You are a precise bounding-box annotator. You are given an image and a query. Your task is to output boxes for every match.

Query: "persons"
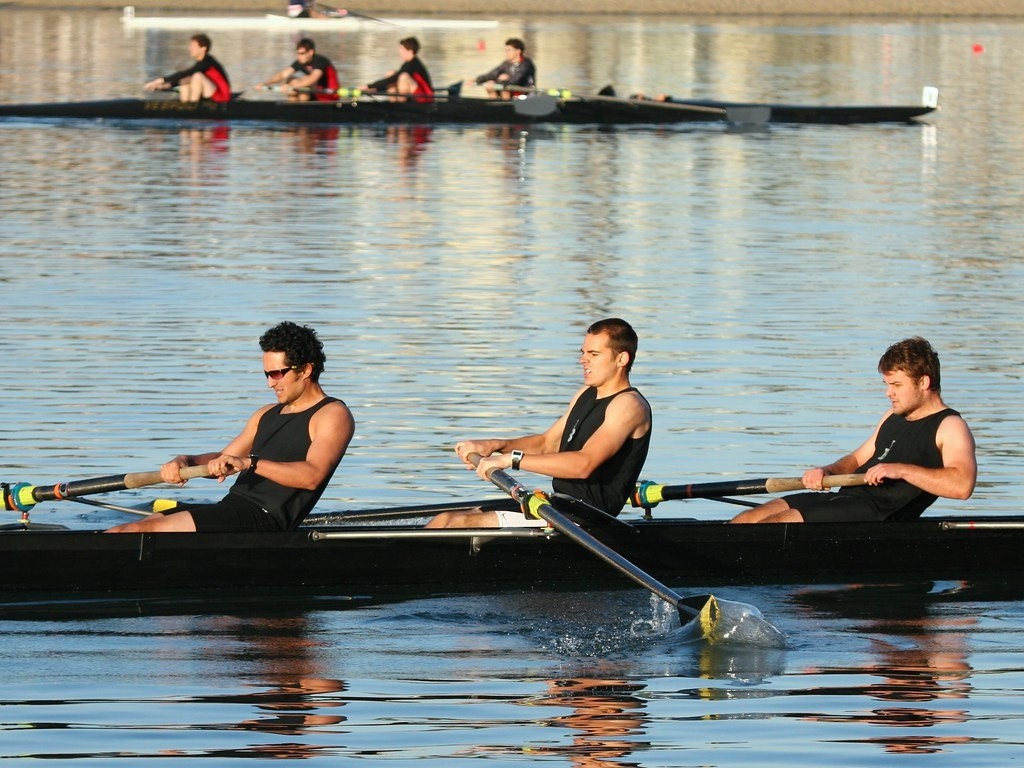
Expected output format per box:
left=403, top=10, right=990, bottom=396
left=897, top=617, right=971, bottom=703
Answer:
left=254, top=37, right=342, bottom=103
left=98, top=319, right=356, bottom=534
left=729, top=336, right=976, bottom=524
left=425, top=318, right=653, bottom=528
left=464, top=37, right=536, bottom=100
left=144, top=34, right=231, bottom=105
left=290, top=1, right=334, bottom=20
left=353, top=37, right=436, bottom=105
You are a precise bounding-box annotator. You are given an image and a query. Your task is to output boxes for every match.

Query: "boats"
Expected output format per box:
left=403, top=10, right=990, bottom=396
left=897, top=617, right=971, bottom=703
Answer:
left=0, top=516, right=1023, bottom=617
left=1, top=87, right=940, bottom=125
left=118, top=6, right=502, bottom=32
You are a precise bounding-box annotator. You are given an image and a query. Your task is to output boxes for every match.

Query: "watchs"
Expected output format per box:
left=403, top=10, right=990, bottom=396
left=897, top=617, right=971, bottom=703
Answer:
left=512, top=450, right=524, bottom=471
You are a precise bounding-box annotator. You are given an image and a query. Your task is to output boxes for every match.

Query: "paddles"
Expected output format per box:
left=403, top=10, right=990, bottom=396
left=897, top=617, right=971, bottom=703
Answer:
left=128, top=473, right=870, bottom=523
left=316, top=2, right=394, bottom=26
left=348, top=79, right=464, bottom=97
left=255, top=84, right=557, bottom=115
left=4, top=464, right=227, bottom=512
left=153, top=86, right=246, bottom=100
left=459, top=446, right=720, bottom=643
left=471, top=81, right=773, bottom=124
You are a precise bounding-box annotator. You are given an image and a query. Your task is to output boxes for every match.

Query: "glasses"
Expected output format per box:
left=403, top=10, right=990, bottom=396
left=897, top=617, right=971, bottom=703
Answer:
left=504, top=48, right=517, bottom=52
left=296, top=51, right=309, bottom=55
left=263, top=362, right=315, bottom=380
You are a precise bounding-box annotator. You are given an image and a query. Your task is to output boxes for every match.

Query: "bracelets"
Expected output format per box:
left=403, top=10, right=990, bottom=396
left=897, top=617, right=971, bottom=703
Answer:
left=247, top=454, right=261, bottom=474
